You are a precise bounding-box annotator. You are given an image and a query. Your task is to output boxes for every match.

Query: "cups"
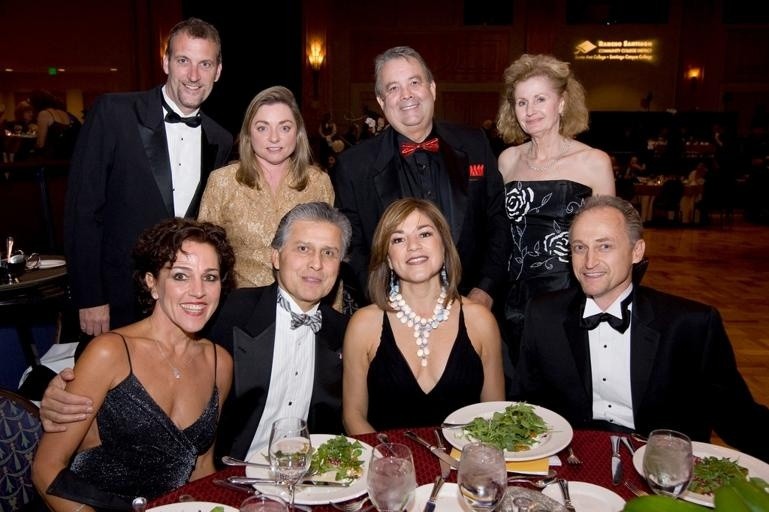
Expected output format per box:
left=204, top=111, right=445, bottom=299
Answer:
left=642, top=429, right=695, bottom=499
left=236, top=492, right=289, bottom=512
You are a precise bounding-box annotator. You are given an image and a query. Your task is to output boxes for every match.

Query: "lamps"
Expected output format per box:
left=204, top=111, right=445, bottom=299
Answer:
left=304, top=30, right=327, bottom=101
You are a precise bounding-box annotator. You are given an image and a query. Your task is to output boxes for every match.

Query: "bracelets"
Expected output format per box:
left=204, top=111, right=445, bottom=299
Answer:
left=75, top=504, right=86, bottom=512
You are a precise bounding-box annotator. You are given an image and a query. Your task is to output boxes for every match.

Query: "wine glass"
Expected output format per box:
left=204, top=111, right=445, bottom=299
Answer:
left=458, top=442, right=509, bottom=512
left=366, top=443, right=417, bottom=512
left=268, top=418, right=313, bottom=512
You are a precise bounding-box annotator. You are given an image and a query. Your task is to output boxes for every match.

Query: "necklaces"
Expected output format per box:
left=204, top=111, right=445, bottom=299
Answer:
left=148, top=316, right=197, bottom=379
left=388, top=284, right=453, bottom=367
left=525, top=135, right=573, bottom=172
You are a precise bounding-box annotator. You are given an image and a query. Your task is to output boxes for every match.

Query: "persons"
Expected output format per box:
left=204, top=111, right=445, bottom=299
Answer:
left=0, top=87, right=89, bottom=185
left=494, top=53, right=618, bottom=356
left=38, top=201, right=351, bottom=472
left=508, top=195, right=768, bottom=466
left=31, top=217, right=234, bottom=512
left=197, top=84, right=336, bottom=290
left=318, top=111, right=385, bottom=172
left=342, top=196, right=506, bottom=437
left=332, top=45, right=512, bottom=312
left=585, top=118, right=769, bottom=229
left=67, top=18, right=234, bottom=371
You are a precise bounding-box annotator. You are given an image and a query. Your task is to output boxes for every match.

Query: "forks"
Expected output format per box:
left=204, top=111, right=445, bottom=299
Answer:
left=566, top=443, right=585, bottom=465
left=329, top=490, right=373, bottom=511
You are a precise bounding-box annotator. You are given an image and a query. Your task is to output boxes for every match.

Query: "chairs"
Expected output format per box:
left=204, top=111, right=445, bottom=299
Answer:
left=1, top=387, right=49, bottom=512
left=614, top=122, right=769, bottom=229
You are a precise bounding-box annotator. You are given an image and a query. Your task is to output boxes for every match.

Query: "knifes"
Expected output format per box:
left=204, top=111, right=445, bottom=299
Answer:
left=623, top=479, right=649, bottom=498
left=433, top=428, right=451, bottom=479
left=558, top=478, right=576, bottom=512
left=608, top=434, right=625, bottom=487
left=423, top=476, right=446, bottom=512
left=377, top=431, right=399, bottom=458
left=404, top=430, right=459, bottom=469
left=227, top=474, right=350, bottom=488
left=212, top=476, right=313, bottom=512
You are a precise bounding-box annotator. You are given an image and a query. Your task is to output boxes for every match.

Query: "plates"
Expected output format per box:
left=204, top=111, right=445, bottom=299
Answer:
left=405, top=483, right=465, bottom=512
left=137, top=501, right=238, bottom=511
left=440, top=401, right=574, bottom=461
left=245, top=433, right=382, bottom=504
left=27, top=258, right=66, bottom=269
left=541, top=480, right=627, bottom=512
left=632, top=438, right=769, bottom=510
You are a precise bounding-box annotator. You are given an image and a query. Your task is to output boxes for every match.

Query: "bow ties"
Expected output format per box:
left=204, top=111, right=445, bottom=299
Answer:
left=291, top=310, right=322, bottom=333
left=165, top=111, right=202, bottom=127
left=400, top=138, right=439, bottom=156
left=580, top=298, right=630, bottom=333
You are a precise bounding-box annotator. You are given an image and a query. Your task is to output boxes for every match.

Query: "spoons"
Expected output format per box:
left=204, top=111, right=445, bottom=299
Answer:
left=509, top=476, right=559, bottom=487
